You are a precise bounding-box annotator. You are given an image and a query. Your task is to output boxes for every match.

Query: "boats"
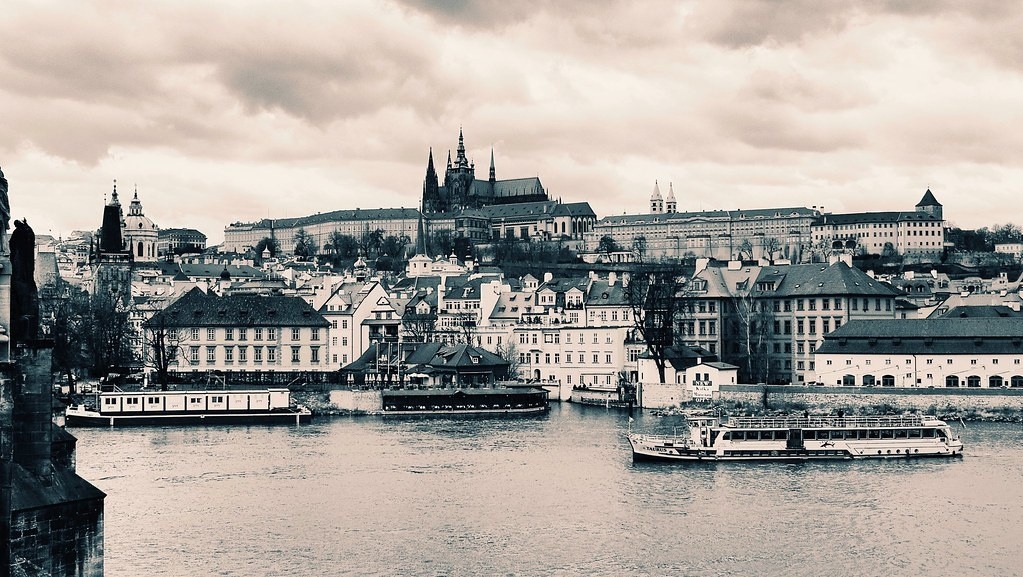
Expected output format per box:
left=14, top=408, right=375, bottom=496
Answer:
left=628, top=407, right=969, bottom=468
left=379, top=385, right=554, bottom=422
